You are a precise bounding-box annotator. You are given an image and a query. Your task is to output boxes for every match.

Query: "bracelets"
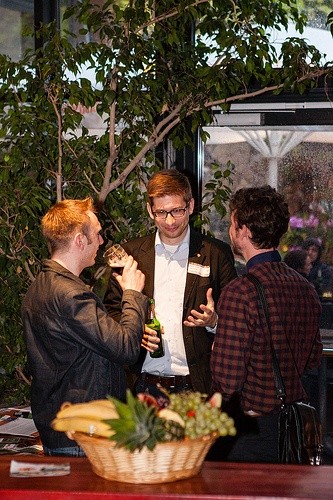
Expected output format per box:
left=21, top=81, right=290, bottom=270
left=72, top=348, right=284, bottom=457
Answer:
left=210, top=322, right=217, bottom=329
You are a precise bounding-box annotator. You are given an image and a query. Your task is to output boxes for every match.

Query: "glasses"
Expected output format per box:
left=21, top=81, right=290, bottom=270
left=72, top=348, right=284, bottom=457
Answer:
left=153, top=204, right=188, bottom=219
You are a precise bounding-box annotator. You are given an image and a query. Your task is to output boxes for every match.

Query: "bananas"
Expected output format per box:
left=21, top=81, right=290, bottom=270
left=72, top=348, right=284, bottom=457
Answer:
left=51, top=399, right=121, bottom=436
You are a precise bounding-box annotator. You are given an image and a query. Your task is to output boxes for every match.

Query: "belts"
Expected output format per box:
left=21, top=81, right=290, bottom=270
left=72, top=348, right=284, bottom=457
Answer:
left=143, top=374, right=191, bottom=388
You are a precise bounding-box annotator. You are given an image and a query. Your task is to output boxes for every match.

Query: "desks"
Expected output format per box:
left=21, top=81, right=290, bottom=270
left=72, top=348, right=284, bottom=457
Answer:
left=0, top=454, right=333, bottom=500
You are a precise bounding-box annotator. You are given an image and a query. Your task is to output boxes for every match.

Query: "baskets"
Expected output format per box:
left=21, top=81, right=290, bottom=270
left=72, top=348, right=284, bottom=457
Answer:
left=61, top=391, right=221, bottom=484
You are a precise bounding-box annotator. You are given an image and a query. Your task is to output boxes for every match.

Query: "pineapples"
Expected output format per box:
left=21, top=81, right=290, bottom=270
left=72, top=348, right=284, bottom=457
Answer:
left=103, top=387, right=186, bottom=452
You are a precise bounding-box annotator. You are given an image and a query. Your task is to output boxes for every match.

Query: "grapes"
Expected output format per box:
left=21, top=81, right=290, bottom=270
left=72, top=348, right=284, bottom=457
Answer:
left=167, top=391, right=237, bottom=440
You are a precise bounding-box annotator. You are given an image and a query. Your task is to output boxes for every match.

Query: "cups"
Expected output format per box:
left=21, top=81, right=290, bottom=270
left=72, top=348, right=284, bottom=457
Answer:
left=102, top=244, right=129, bottom=276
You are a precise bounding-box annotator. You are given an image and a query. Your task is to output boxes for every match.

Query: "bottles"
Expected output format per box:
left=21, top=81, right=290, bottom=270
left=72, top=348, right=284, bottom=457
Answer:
left=144, top=298, right=165, bottom=358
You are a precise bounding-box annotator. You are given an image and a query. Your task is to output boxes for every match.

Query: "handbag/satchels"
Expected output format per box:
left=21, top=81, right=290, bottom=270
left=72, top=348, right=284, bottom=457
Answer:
left=278, top=403, right=324, bottom=466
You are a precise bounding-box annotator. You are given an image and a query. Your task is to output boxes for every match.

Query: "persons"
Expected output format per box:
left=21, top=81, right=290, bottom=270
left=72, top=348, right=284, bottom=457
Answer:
left=103, top=169, right=240, bottom=462
left=209, top=185, right=323, bottom=466
left=21, top=196, right=149, bottom=457
left=285, top=239, right=329, bottom=296
